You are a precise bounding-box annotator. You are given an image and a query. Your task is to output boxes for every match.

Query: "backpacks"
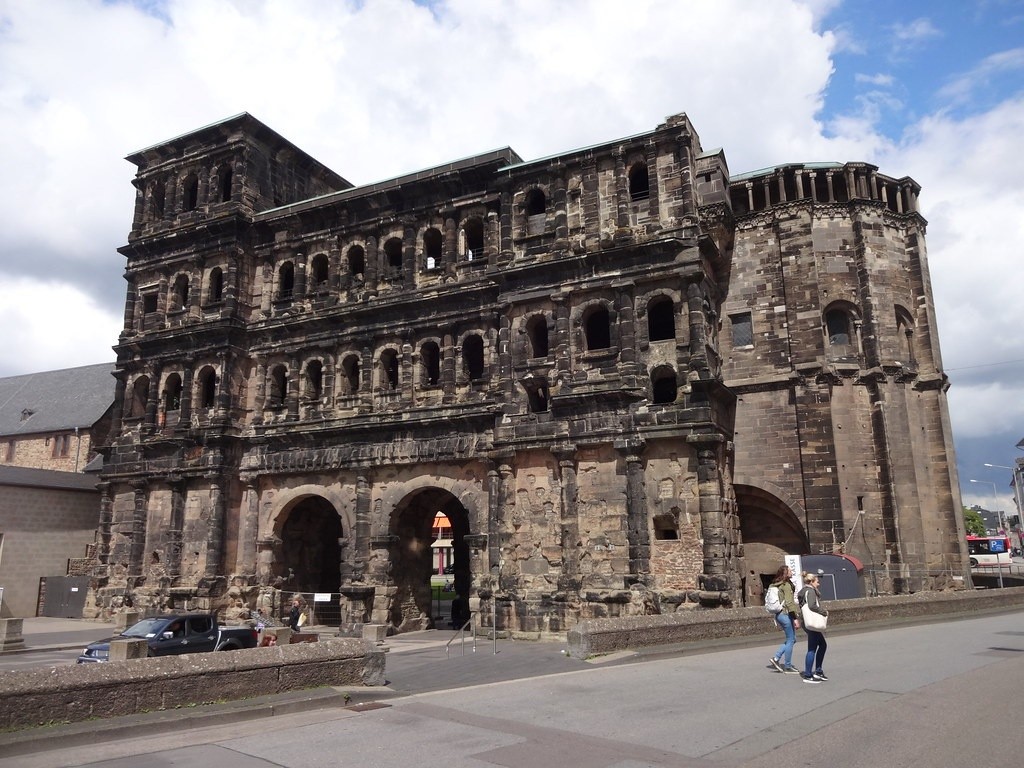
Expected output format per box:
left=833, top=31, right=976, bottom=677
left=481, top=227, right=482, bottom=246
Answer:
left=765, top=587, right=793, bottom=613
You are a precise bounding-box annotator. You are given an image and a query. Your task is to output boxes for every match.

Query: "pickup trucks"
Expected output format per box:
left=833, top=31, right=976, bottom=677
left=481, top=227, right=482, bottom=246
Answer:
left=77, top=610, right=257, bottom=665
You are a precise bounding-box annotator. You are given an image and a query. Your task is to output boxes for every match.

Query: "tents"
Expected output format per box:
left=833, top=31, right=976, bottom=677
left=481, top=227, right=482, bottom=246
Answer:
left=801, top=553, right=866, bottom=600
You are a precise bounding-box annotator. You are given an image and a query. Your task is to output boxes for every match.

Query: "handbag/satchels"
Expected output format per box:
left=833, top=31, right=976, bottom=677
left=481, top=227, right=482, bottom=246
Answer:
left=801, top=590, right=829, bottom=632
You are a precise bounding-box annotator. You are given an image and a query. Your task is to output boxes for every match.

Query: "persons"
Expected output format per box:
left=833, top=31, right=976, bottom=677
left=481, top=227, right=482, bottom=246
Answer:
left=173, top=619, right=184, bottom=639
left=258, top=634, right=277, bottom=647
left=171, top=397, right=179, bottom=411
left=797, top=570, right=829, bottom=683
left=769, top=564, right=800, bottom=675
left=288, top=595, right=303, bottom=634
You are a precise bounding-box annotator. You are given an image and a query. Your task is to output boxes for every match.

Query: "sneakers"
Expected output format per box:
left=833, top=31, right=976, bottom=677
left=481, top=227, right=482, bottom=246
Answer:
left=783, top=666, right=800, bottom=675
left=770, top=657, right=783, bottom=673
left=802, top=675, right=821, bottom=684
left=813, top=671, right=828, bottom=681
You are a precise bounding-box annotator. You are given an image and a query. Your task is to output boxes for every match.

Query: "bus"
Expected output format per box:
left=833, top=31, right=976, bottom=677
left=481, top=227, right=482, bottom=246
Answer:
left=966, top=534, right=1013, bottom=569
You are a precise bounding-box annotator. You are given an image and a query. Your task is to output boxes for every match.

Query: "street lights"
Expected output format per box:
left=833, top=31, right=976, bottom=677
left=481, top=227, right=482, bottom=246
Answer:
left=969, top=479, right=1005, bottom=535
left=983, top=462, right=1024, bottom=529
